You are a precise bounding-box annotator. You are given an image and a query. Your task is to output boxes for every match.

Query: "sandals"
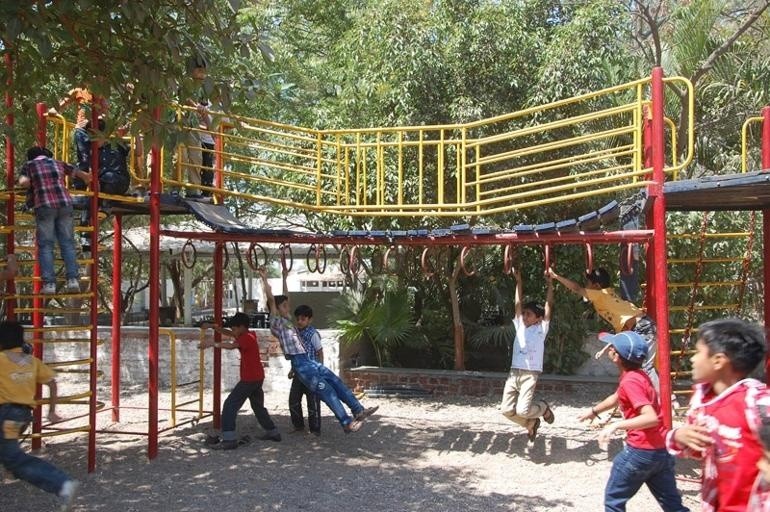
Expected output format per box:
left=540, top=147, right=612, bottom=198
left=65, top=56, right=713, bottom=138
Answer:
left=527, top=418, right=540, bottom=442
left=540, top=400, right=554, bottom=424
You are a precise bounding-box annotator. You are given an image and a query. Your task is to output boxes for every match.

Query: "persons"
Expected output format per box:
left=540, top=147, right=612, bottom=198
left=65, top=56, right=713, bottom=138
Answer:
left=574, top=330, right=691, bottom=512
left=196, top=311, right=282, bottom=451
left=614, top=192, right=646, bottom=305
left=18, top=145, right=102, bottom=296
left=543, top=265, right=681, bottom=419
left=500, top=262, right=555, bottom=442
left=49, top=82, right=135, bottom=190
left=194, top=108, right=217, bottom=198
left=0, top=319, right=82, bottom=512
left=664, top=317, right=769, bottom=512
left=285, top=304, right=324, bottom=439
left=72, top=119, right=132, bottom=253
left=170, top=57, right=216, bottom=197
left=258, top=263, right=380, bottom=435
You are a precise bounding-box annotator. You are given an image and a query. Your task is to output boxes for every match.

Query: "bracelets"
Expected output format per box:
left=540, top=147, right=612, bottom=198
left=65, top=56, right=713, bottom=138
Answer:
left=592, top=407, right=602, bottom=420
left=214, top=341, right=219, bottom=348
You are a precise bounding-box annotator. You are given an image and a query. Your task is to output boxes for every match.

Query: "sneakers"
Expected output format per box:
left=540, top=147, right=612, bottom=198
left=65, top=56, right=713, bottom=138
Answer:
left=65, top=278, right=79, bottom=288
left=305, top=432, right=320, bottom=440
left=343, top=421, right=363, bottom=432
left=209, top=440, right=238, bottom=450
left=258, top=433, right=281, bottom=442
left=60, top=480, right=81, bottom=511
left=289, top=429, right=304, bottom=435
left=40, top=283, right=55, bottom=294
left=356, top=405, right=379, bottom=420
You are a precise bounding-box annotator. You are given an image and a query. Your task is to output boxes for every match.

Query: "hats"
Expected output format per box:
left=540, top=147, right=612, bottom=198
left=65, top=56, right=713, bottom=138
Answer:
left=598, top=331, right=648, bottom=364
